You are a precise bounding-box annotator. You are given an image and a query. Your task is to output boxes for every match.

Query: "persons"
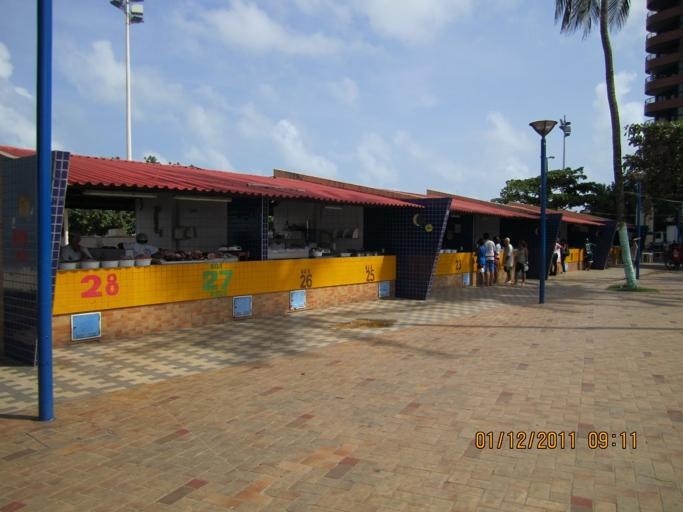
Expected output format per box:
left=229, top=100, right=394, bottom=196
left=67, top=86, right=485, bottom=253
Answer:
left=511, top=239, right=528, bottom=287
left=503, top=237, right=513, bottom=283
left=482, top=231, right=495, bottom=287
left=646, top=240, right=682, bottom=270
left=117, top=232, right=158, bottom=258
left=473, top=238, right=485, bottom=286
left=59, top=231, right=93, bottom=262
left=548, top=236, right=569, bottom=275
left=493, top=236, right=500, bottom=284
left=579, top=237, right=595, bottom=271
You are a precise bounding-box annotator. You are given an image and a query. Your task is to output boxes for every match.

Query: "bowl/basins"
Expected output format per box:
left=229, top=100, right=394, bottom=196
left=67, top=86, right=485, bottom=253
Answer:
left=79, top=261, right=99, bottom=269
left=118, top=259, right=135, bottom=267
left=439, top=249, right=457, bottom=254
left=339, top=252, right=352, bottom=257
left=135, top=259, right=152, bottom=266
left=100, top=261, right=119, bottom=267
left=312, top=252, right=322, bottom=257
left=58, top=262, right=76, bottom=270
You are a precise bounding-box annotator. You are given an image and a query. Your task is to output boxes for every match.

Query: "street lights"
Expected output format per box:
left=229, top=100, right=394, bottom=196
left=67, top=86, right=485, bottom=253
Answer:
left=559, top=116, right=571, bottom=169
left=528, top=120, right=558, bottom=304
left=631, top=172, right=648, bottom=280
left=111, top=0, right=144, bottom=161
